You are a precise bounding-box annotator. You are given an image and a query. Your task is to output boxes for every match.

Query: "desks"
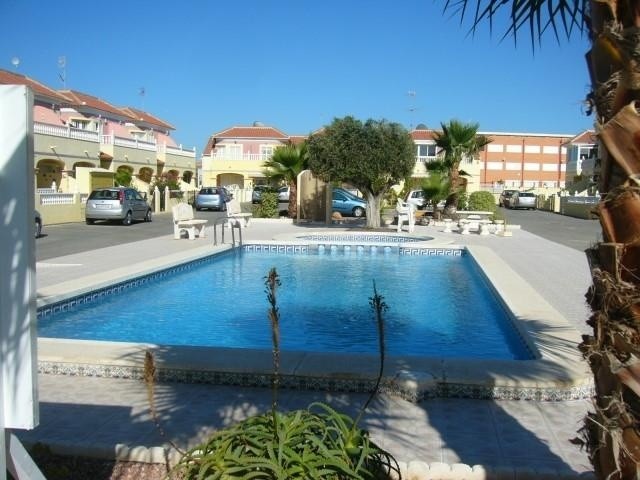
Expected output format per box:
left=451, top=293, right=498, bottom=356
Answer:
left=455, top=210, right=494, bottom=231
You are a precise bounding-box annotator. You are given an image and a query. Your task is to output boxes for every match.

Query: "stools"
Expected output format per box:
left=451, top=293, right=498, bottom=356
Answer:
left=443, top=219, right=504, bottom=235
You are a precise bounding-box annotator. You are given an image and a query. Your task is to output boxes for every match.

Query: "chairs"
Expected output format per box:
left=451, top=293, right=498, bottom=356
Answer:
left=171, top=203, right=208, bottom=240
left=226, top=198, right=253, bottom=228
left=396, top=198, right=415, bottom=234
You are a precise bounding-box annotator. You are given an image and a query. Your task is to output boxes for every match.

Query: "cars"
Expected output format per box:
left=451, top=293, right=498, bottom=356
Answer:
left=406, top=190, right=469, bottom=211
left=85, top=187, right=151, bottom=225
left=196, top=187, right=233, bottom=212
left=35, top=211, right=42, bottom=239
left=331, top=188, right=367, bottom=217
left=276, top=185, right=290, bottom=201
left=252, top=185, right=273, bottom=204
left=508, top=191, right=537, bottom=210
left=499, top=190, right=519, bottom=208
